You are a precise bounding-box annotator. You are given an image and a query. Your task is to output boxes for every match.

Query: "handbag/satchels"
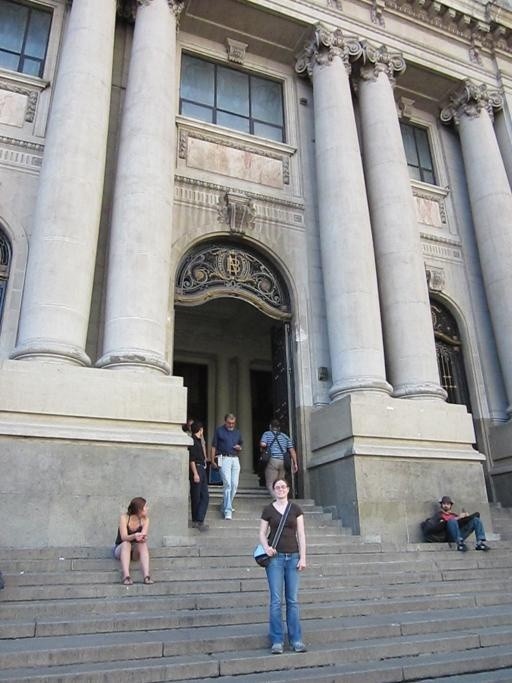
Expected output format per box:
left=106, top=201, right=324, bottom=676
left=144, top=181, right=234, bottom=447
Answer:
left=253, top=545, right=269, bottom=566
left=284, top=452, right=291, bottom=472
left=421, top=518, right=446, bottom=542
left=260, top=449, right=270, bottom=465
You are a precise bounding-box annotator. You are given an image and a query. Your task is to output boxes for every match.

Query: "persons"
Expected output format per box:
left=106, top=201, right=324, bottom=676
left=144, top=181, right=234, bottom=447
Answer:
left=113, top=496, right=156, bottom=585
left=188, top=420, right=210, bottom=532
left=257, top=477, right=307, bottom=655
left=210, top=411, right=244, bottom=520
left=437, top=495, right=491, bottom=552
left=184, top=418, right=208, bottom=462
left=258, top=418, right=299, bottom=499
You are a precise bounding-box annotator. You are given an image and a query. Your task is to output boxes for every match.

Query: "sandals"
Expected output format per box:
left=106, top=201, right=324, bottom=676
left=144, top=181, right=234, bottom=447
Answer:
left=123, top=577, right=132, bottom=585
left=143, top=576, right=153, bottom=584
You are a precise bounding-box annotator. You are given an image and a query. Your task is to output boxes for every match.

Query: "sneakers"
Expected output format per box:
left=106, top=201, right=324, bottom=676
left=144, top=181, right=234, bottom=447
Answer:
left=457, top=543, right=468, bottom=552
left=225, top=515, right=231, bottom=520
left=271, top=641, right=284, bottom=654
left=289, top=642, right=306, bottom=652
left=475, top=542, right=489, bottom=550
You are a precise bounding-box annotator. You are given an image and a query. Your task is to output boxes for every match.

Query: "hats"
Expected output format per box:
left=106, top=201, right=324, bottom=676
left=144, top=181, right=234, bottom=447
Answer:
left=439, top=496, right=454, bottom=504
